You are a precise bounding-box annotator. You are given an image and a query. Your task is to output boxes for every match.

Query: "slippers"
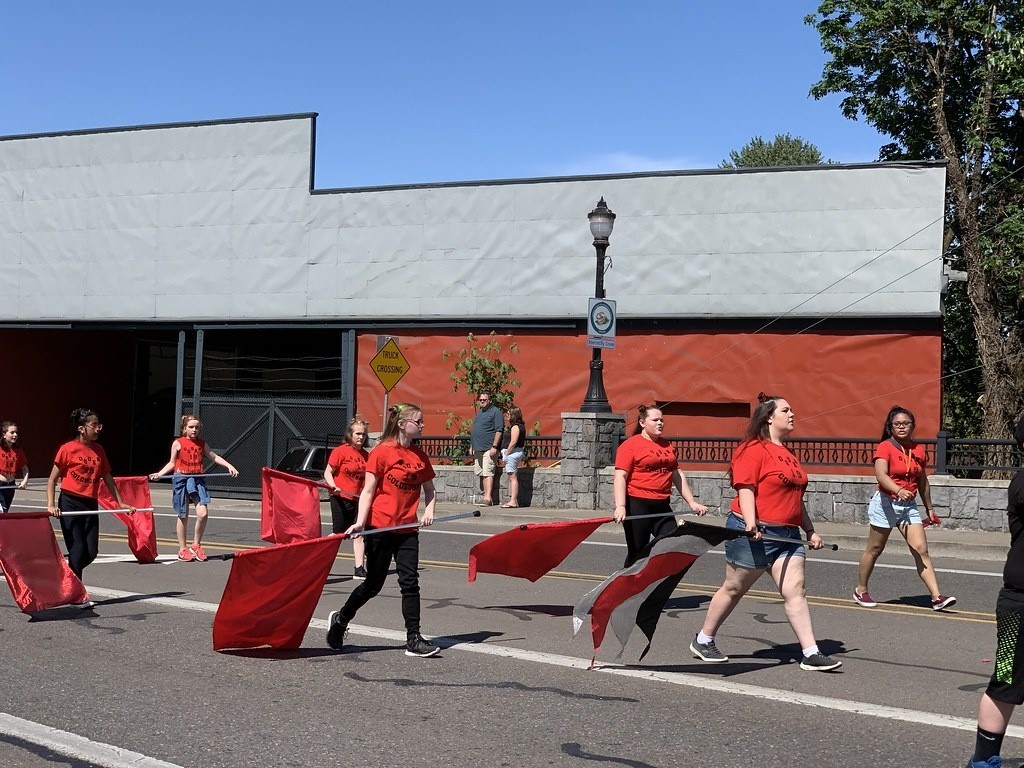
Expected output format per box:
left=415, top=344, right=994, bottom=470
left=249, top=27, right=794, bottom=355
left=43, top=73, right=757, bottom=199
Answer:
left=500, top=502, right=519, bottom=508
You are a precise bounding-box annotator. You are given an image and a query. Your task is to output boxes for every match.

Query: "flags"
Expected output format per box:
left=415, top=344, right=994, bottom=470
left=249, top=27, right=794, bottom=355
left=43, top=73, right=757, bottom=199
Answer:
left=260, top=468, right=335, bottom=545
left=0, top=512, right=90, bottom=614
left=467, top=517, right=612, bottom=585
left=98, top=476, right=158, bottom=564
left=570, top=521, right=756, bottom=672
left=213, top=533, right=346, bottom=653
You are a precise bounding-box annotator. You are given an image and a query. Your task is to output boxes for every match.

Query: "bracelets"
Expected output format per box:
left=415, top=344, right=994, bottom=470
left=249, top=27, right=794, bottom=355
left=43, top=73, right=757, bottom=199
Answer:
left=927, top=508, right=933, bottom=511
left=615, top=505, right=626, bottom=510
left=896, top=486, right=904, bottom=494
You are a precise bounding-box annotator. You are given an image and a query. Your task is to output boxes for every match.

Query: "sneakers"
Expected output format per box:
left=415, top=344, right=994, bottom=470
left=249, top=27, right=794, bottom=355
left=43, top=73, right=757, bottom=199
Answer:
left=931, top=595, right=956, bottom=611
left=853, top=586, right=877, bottom=607
left=800, top=651, right=842, bottom=670
left=689, top=632, right=729, bottom=662
left=326, top=611, right=347, bottom=651
left=70, top=601, right=93, bottom=609
left=404, top=633, right=440, bottom=657
left=177, top=544, right=207, bottom=562
left=353, top=564, right=367, bottom=580
left=965, top=755, right=1002, bottom=768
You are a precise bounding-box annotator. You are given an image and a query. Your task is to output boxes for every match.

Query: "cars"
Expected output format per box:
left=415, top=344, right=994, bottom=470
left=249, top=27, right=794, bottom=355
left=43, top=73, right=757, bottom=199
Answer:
left=275, top=446, right=335, bottom=481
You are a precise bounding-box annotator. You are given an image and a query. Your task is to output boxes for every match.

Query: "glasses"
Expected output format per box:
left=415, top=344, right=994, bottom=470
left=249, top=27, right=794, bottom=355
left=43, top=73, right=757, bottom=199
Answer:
left=84, top=423, right=103, bottom=431
left=402, top=418, right=423, bottom=425
left=890, top=420, right=913, bottom=427
left=479, top=399, right=488, bottom=401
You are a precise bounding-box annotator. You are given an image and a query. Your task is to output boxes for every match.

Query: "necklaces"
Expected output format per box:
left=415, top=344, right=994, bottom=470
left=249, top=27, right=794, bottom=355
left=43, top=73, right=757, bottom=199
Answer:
left=892, top=436, right=912, bottom=481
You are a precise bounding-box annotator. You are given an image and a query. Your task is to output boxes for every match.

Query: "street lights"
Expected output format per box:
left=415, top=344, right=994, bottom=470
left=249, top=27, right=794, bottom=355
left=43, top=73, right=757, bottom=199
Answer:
left=578, top=195, right=618, bottom=412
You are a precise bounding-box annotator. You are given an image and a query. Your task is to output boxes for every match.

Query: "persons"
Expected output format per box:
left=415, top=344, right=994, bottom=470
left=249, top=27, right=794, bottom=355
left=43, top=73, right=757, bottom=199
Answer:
left=689, top=391, right=843, bottom=671
left=149, top=415, right=238, bottom=562
left=613, top=404, right=709, bottom=569
left=469, top=391, right=526, bottom=509
left=852, top=405, right=957, bottom=611
left=0, top=421, right=29, bottom=574
left=47, top=407, right=136, bottom=609
left=326, top=403, right=441, bottom=657
left=324, top=419, right=370, bottom=580
left=965, top=417, right=1024, bottom=768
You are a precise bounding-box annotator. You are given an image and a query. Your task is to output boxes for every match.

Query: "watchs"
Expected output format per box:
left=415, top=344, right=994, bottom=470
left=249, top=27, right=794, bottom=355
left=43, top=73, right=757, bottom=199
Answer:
left=492, top=446, right=498, bottom=448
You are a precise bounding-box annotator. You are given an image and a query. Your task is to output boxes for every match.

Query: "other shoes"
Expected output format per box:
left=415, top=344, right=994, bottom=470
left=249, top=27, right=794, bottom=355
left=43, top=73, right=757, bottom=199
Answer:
left=474, top=498, right=493, bottom=507
